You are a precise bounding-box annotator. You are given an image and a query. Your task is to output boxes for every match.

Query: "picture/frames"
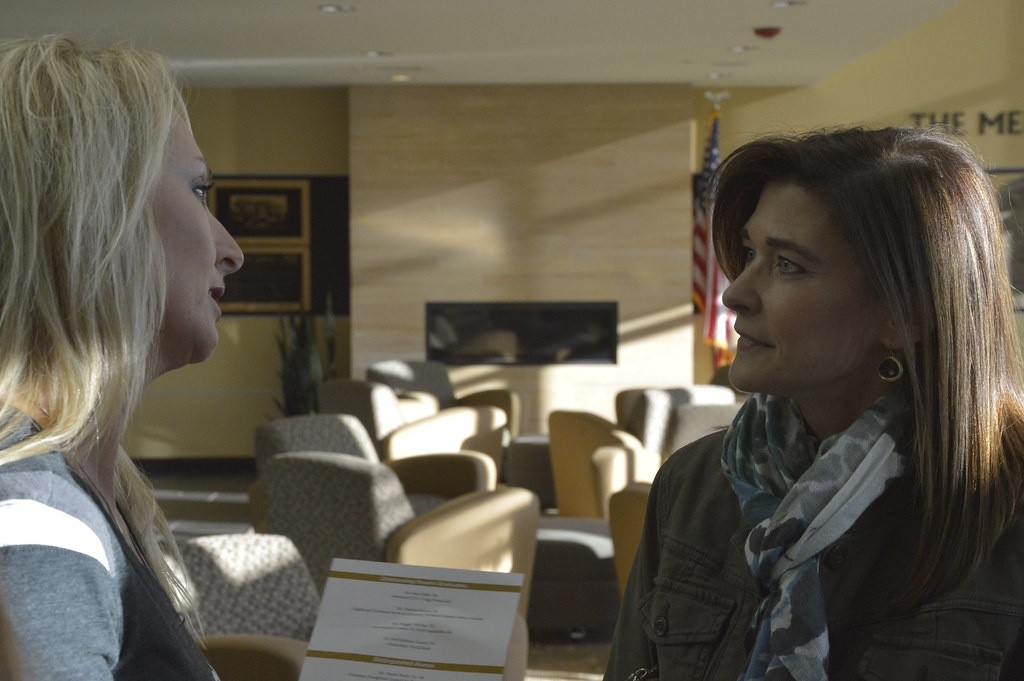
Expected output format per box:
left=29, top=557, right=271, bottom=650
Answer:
left=217, top=246, right=311, bottom=313
left=207, top=180, right=311, bottom=246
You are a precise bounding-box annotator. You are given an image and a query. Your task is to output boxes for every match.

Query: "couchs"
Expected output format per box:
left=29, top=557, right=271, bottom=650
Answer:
left=317, top=379, right=407, bottom=462
left=386, top=487, right=540, bottom=617
left=249, top=449, right=416, bottom=596
left=615, top=385, right=734, bottom=432
left=549, top=410, right=654, bottom=520
left=253, top=413, right=450, bottom=517
left=367, top=358, right=523, bottom=436
left=383, top=404, right=507, bottom=472
left=177, top=530, right=319, bottom=641
left=608, top=482, right=652, bottom=597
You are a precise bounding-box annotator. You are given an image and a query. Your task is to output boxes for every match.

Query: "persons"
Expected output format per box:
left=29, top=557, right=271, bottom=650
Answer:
left=602, top=126, right=1024, bottom=680
left=0, top=34, right=244, bottom=681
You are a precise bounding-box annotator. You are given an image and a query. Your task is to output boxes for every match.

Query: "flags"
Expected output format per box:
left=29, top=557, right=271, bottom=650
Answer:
left=693, top=117, right=741, bottom=370
